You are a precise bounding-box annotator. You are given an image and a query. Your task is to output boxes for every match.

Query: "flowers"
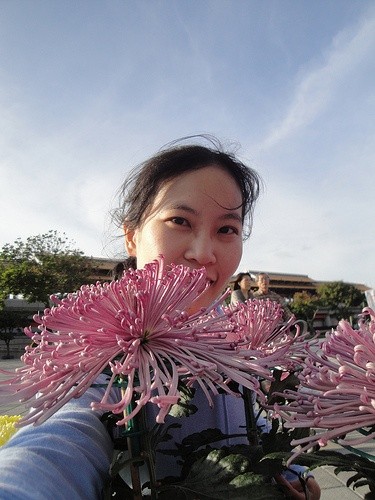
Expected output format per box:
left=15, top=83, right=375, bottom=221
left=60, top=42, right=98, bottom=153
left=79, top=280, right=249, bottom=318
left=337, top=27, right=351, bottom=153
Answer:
left=1, top=254, right=375, bottom=500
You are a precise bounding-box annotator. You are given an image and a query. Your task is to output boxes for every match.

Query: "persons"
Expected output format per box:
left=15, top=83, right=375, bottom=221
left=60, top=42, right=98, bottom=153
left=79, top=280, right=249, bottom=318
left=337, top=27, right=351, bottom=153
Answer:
left=0, top=145, right=323, bottom=500
left=229, top=271, right=257, bottom=306
left=252, top=272, right=301, bottom=336
